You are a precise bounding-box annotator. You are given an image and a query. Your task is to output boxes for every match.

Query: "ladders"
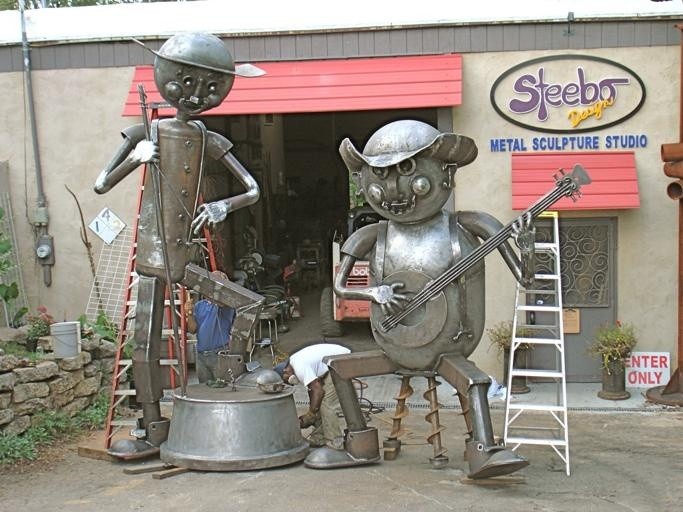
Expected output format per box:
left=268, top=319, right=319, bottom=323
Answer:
left=104, top=102, right=215, bottom=451
left=503, top=211, right=571, bottom=476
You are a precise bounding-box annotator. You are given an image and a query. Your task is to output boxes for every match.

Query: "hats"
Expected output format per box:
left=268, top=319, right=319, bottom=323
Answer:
left=273, top=352, right=290, bottom=376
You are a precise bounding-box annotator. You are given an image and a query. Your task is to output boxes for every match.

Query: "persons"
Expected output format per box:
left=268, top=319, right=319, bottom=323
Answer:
left=182, top=270, right=236, bottom=383
left=275, top=342, right=355, bottom=450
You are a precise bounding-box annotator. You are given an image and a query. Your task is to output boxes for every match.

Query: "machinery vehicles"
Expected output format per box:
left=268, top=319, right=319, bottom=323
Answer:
left=318, top=204, right=388, bottom=341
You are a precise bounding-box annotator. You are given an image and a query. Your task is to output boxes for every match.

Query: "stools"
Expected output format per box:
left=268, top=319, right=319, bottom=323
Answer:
left=249, top=311, right=279, bottom=362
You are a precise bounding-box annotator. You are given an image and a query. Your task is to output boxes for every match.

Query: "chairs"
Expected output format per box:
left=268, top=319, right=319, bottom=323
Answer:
left=244, top=270, right=295, bottom=333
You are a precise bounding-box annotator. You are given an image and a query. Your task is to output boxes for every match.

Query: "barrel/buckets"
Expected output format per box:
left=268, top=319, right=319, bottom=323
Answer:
left=55, top=321, right=82, bottom=354
left=50, top=324, right=80, bottom=360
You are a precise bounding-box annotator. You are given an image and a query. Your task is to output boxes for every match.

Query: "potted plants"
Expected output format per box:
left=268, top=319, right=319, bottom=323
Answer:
left=485, top=320, right=540, bottom=394
left=579, top=319, right=640, bottom=401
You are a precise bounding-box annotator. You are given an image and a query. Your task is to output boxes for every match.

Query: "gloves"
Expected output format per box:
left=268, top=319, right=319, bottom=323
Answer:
left=299, top=411, right=318, bottom=429
